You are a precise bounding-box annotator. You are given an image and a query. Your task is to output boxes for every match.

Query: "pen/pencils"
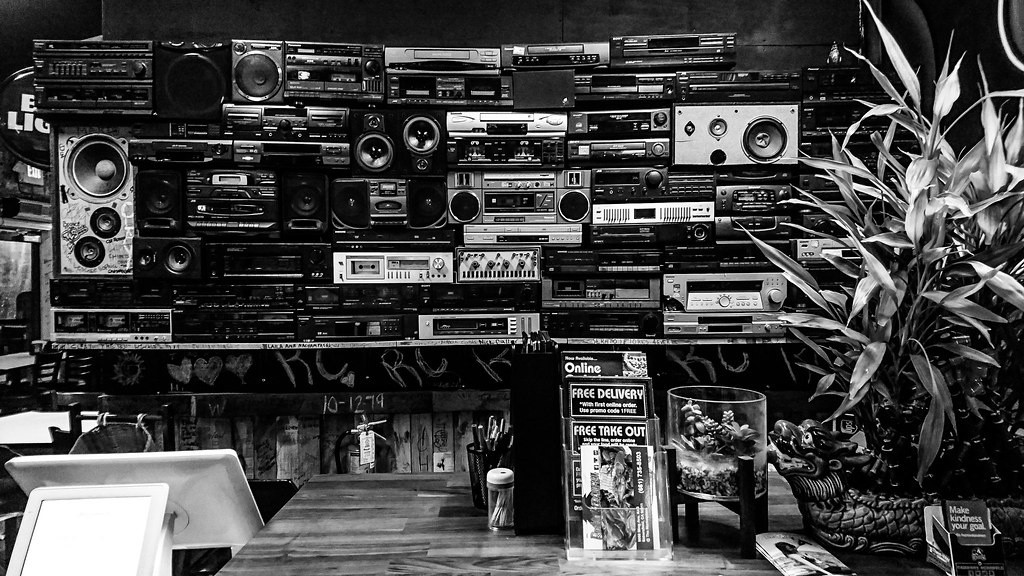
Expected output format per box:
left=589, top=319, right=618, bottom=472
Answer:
left=471, top=415, right=513, bottom=451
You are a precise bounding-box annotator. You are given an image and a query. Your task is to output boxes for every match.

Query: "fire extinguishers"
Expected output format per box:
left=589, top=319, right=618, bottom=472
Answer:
left=333, top=415, right=388, bottom=474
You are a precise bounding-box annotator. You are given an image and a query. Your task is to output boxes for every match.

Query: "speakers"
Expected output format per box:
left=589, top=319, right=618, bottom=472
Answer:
left=57, top=35, right=801, bottom=281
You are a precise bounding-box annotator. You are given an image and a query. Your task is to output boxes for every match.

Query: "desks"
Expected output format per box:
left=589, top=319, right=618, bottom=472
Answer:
left=214, top=473, right=802, bottom=576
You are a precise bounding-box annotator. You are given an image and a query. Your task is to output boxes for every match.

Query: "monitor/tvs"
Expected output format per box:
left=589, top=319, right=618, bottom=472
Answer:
left=6, top=482, right=169, bottom=576
left=4, top=449, right=265, bottom=560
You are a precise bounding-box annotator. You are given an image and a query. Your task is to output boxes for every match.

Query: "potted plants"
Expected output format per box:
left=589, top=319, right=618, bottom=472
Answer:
left=734, top=0, right=1024, bottom=553
